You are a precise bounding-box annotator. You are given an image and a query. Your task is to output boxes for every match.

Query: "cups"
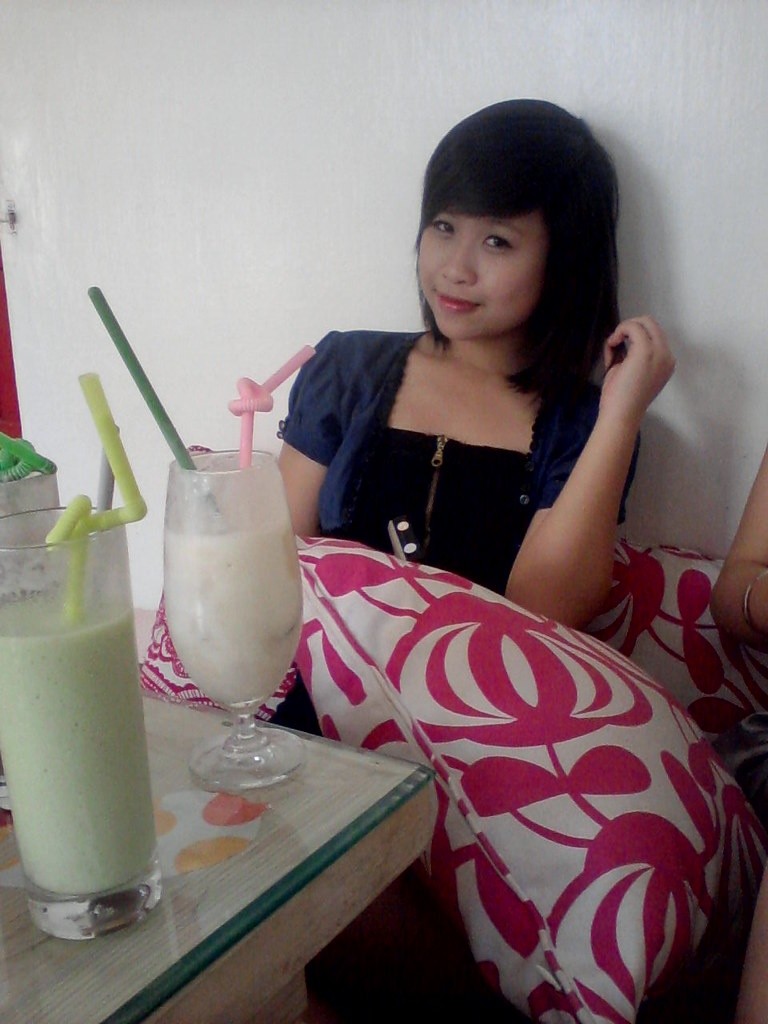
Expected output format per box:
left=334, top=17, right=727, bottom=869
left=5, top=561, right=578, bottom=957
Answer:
left=0, top=469, right=166, bottom=942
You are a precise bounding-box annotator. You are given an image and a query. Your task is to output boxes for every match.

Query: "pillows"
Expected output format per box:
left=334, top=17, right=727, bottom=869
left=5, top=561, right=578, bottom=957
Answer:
left=141, top=446, right=768, bottom=1024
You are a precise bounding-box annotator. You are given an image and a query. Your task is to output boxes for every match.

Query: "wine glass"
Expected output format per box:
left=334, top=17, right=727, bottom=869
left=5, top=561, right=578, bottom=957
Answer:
left=163, top=449, right=304, bottom=791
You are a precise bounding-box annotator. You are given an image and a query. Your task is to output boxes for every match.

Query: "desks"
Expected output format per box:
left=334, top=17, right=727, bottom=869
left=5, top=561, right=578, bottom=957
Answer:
left=0, top=694, right=439, bottom=1024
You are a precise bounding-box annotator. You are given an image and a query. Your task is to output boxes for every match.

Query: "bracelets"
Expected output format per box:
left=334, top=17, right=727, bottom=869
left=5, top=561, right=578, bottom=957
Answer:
left=743, top=570, right=768, bottom=635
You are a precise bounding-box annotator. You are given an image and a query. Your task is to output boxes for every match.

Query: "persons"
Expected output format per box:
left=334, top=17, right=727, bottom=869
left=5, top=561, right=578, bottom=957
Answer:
left=270, top=100, right=674, bottom=1024
left=710, top=442, right=768, bottom=1023
